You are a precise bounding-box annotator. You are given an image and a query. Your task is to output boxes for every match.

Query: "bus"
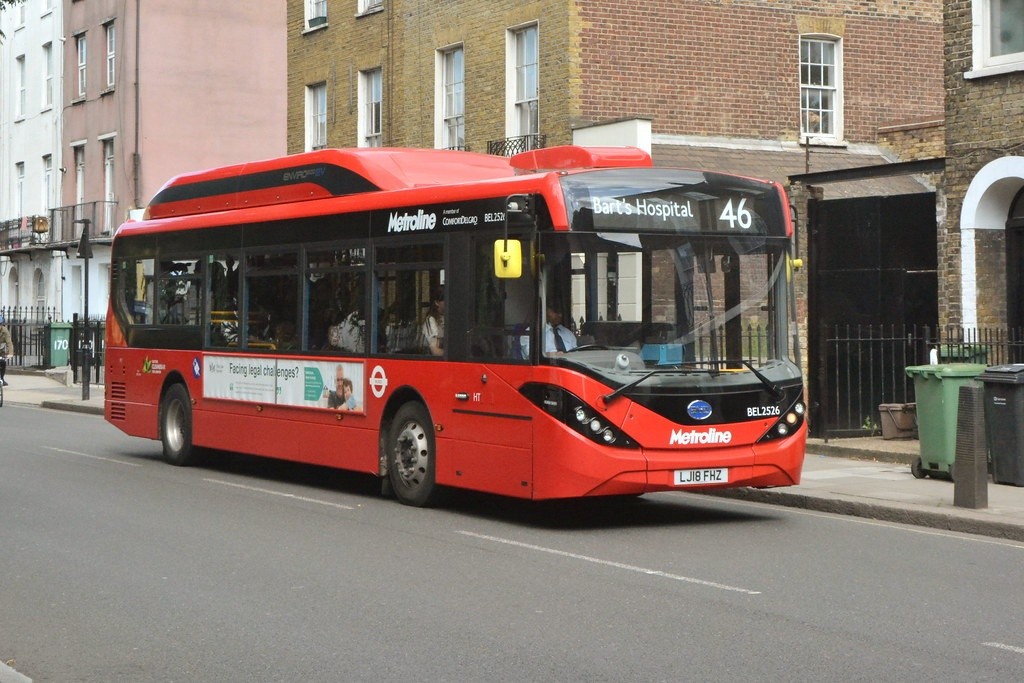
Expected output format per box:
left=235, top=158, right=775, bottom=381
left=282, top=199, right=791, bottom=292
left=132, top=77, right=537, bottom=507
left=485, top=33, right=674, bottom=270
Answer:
left=103, top=146, right=808, bottom=508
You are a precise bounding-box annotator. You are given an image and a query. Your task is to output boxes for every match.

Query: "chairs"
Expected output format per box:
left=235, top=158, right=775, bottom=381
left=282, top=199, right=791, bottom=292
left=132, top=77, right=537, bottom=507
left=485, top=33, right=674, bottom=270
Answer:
left=510, top=323, right=522, bottom=361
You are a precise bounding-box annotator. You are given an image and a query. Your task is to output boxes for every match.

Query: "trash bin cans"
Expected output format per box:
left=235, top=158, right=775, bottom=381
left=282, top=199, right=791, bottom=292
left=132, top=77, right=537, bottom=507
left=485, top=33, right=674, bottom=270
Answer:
left=905, top=362, right=990, bottom=482
left=974, top=363, right=1024, bottom=487
left=42, top=322, right=73, bottom=368
left=71, top=322, right=106, bottom=366
left=936, top=344, right=990, bottom=364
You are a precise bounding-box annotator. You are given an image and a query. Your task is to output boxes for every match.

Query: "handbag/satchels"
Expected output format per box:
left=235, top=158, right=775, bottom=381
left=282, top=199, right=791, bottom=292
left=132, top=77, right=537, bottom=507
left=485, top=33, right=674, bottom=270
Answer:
left=329, top=313, right=365, bottom=353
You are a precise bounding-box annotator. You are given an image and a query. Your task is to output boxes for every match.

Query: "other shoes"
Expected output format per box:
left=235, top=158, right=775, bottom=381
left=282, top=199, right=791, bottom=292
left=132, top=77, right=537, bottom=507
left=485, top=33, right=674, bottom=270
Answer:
left=3, top=380, right=8, bottom=386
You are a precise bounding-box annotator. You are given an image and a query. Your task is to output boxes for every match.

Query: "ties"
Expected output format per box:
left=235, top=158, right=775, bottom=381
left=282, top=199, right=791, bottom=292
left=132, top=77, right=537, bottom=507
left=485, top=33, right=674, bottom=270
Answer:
left=553, top=328, right=567, bottom=353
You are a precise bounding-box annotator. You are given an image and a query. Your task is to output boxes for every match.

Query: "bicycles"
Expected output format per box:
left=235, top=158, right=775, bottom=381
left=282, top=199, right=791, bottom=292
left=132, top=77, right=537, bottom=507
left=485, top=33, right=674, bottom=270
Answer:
left=0, top=355, right=12, bottom=407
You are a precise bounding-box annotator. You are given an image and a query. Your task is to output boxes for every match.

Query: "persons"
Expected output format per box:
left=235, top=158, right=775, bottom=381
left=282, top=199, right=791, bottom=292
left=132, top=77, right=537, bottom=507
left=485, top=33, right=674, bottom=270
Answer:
left=519, top=295, right=577, bottom=358
left=142, top=257, right=446, bottom=356
left=0, top=316, right=13, bottom=386
